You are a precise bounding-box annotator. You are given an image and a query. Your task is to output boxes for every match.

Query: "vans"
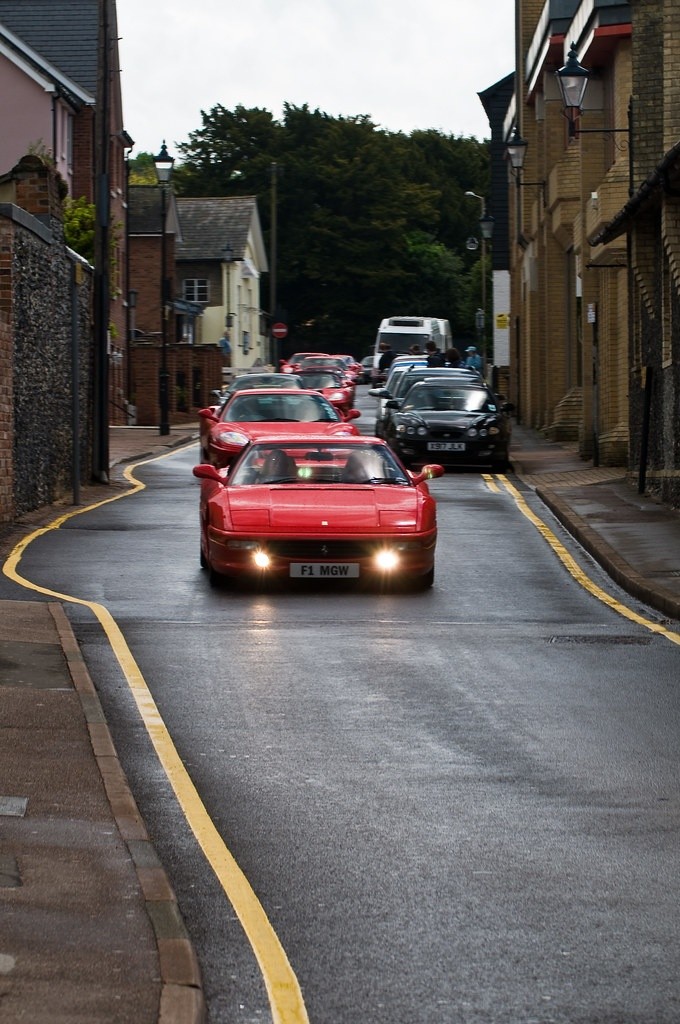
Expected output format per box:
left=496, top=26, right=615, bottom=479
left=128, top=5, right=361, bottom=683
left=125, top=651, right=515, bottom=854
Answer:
left=372, top=316, right=454, bottom=380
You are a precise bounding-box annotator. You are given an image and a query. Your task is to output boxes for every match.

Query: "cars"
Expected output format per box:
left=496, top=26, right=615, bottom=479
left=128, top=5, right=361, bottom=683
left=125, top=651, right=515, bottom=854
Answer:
left=361, top=354, right=374, bottom=381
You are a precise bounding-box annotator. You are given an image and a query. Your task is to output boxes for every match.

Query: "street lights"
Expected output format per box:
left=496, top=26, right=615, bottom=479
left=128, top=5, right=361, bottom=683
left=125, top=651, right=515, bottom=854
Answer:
left=465, top=189, right=490, bottom=379
left=154, top=140, right=173, bottom=439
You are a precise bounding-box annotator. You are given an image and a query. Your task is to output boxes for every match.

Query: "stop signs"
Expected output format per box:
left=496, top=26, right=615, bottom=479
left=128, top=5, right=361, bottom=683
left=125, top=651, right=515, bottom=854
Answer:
left=272, top=322, right=288, bottom=338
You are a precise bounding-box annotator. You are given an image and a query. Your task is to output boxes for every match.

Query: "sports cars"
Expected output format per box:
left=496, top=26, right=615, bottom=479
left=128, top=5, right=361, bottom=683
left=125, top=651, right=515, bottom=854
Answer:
left=369, top=352, right=507, bottom=442
left=192, top=433, right=445, bottom=593
left=196, top=388, right=363, bottom=472
left=280, top=351, right=365, bottom=418
left=211, top=373, right=302, bottom=404
left=377, top=377, right=517, bottom=473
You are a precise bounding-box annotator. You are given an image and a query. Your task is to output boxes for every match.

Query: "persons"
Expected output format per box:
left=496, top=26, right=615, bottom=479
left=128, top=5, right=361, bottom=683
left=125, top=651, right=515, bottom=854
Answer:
left=343, top=451, right=366, bottom=480
left=219, top=332, right=232, bottom=353
left=425, top=341, right=445, bottom=368
left=240, top=399, right=259, bottom=419
left=411, top=345, right=425, bottom=355
left=255, top=450, right=289, bottom=484
left=446, top=348, right=468, bottom=369
left=373, top=343, right=397, bottom=388
left=465, top=346, right=481, bottom=372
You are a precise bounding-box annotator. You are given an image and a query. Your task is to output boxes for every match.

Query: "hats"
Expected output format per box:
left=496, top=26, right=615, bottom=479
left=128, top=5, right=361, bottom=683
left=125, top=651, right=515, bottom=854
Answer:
left=465, top=346, right=477, bottom=352
